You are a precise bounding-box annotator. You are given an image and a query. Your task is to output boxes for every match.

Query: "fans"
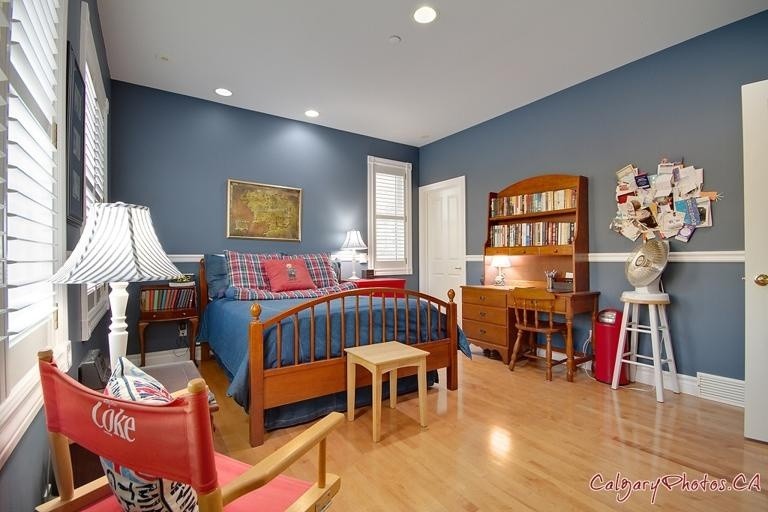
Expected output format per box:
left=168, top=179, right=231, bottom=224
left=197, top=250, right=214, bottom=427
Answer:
left=622, top=239, right=669, bottom=302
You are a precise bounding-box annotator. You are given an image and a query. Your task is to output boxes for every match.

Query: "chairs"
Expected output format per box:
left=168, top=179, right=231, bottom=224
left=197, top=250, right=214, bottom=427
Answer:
left=35, top=349, right=346, bottom=512
left=509, top=287, right=577, bottom=381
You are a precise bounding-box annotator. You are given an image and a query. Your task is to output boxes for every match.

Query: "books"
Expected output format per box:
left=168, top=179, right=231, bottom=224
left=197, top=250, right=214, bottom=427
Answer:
left=142, top=289, right=193, bottom=310
left=489, top=188, right=577, bottom=248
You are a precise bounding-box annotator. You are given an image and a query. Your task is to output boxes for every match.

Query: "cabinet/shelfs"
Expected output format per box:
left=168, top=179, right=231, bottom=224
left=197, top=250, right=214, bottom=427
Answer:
left=459, top=175, right=601, bottom=382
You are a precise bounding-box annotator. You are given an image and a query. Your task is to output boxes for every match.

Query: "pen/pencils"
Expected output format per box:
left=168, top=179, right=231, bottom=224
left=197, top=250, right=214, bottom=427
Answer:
left=544, top=265, right=558, bottom=278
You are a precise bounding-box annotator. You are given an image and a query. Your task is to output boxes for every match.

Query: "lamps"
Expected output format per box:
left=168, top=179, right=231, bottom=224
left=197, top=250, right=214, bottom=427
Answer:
left=48, top=201, right=184, bottom=374
left=340, top=230, right=368, bottom=280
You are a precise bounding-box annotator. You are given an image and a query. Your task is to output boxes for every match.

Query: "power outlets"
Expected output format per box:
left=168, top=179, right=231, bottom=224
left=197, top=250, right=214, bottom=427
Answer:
left=178, top=324, right=187, bottom=337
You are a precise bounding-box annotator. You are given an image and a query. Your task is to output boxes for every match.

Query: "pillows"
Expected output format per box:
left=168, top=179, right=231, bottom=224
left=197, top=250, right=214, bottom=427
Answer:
left=99, top=356, right=198, bottom=512
left=204, top=250, right=358, bottom=301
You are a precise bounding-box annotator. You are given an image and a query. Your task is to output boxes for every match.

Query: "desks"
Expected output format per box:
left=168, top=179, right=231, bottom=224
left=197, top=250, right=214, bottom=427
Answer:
left=139, top=361, right=219, bottom=431
left=611, top=298, right=680, bottom=403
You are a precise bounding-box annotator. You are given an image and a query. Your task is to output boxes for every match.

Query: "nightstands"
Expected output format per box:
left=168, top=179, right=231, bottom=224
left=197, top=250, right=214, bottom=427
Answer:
left=137, top=285, right=199, bottom=367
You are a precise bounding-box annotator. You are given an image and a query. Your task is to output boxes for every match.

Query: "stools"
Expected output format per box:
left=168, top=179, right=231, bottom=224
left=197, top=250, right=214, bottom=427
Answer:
left=343, top=340, right=431, bottom=442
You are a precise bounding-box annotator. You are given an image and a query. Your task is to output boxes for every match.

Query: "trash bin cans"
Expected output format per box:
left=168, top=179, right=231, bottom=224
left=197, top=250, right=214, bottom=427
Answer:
left=592, top=308, right=630, bottom=386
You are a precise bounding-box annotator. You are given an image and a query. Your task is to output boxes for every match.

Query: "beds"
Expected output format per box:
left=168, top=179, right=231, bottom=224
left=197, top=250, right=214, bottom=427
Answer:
left=198, top=258, right=457, bottom=448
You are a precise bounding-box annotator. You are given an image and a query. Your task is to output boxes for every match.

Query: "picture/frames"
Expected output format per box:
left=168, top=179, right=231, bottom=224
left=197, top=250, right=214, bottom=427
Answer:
left=227, top=179, right=302, bottom=242
